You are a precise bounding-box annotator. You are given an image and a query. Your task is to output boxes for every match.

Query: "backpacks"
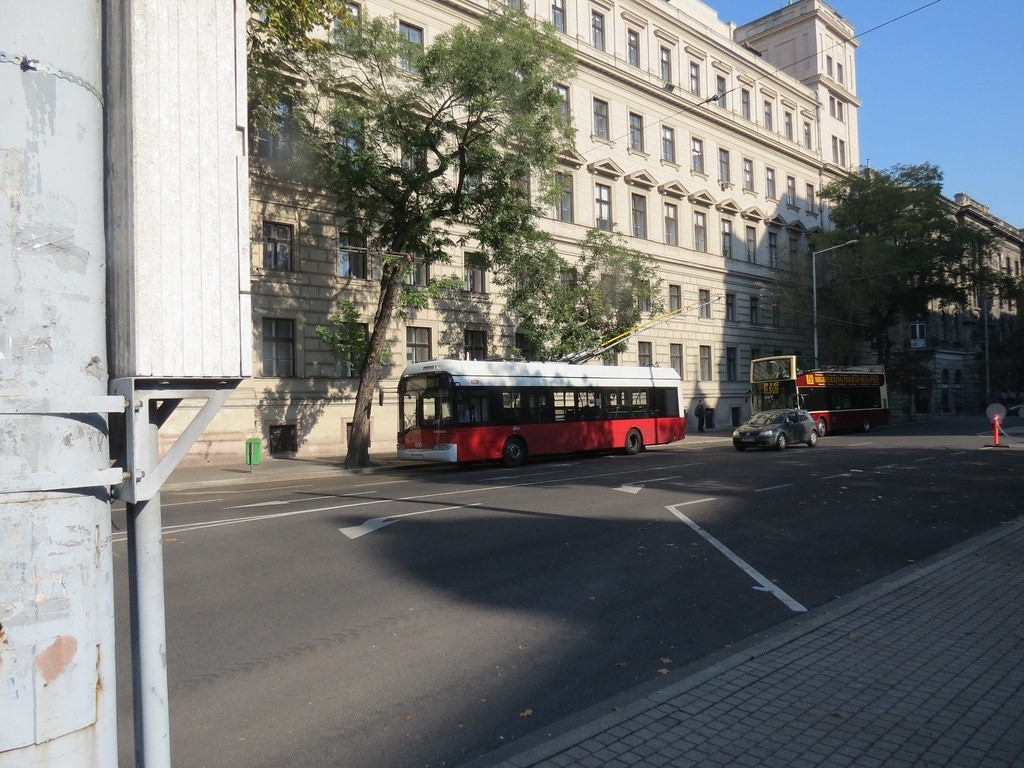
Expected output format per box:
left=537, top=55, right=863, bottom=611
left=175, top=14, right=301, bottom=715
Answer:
left=694, top=404, right=700, bottom=416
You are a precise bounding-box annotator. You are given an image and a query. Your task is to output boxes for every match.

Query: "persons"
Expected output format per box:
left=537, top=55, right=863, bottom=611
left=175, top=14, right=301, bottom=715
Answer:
left=695, top=400, right=705, bottom=433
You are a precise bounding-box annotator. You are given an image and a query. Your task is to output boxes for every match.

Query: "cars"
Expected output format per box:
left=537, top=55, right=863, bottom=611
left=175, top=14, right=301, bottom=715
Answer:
left=731, top=408, right=820, bottom=452
left=1006, top=402, right=1024, bottom=418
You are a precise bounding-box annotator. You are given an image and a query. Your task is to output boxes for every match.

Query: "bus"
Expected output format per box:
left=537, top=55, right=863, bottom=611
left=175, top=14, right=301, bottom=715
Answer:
left=379, top=294, right=721, bottom=469
left=743, top=356, right=890, bottom=435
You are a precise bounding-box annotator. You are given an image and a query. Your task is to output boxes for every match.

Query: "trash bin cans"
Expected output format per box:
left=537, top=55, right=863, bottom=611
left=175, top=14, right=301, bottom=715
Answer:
left=245, top=437, right=262, bottom=465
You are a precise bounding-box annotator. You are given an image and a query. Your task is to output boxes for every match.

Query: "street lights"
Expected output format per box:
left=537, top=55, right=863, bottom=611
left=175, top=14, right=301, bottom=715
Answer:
left=811, top=239, right=858, bottom=369
